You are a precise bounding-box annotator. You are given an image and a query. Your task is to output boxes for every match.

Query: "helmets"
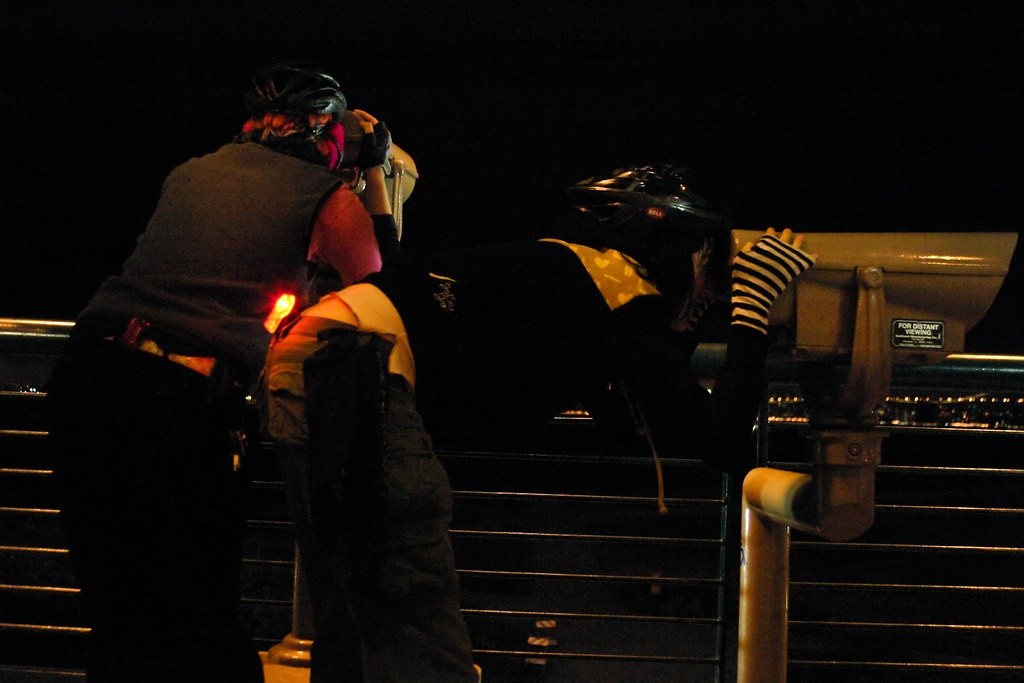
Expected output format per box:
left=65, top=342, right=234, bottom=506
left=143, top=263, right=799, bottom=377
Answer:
left=577, top=164, right=729, bottom=224
left=238, top=65, right=347, bottom=122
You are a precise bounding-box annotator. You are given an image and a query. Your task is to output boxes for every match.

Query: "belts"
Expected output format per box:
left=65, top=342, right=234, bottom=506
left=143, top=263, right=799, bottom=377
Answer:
left=105, top=335, right=217, bottom=377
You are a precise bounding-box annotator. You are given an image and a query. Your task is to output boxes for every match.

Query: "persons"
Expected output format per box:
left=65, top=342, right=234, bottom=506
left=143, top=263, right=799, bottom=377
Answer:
left=263, top=163, right=820, bottom=683
left=44, top=64, right=402, bottom=682
